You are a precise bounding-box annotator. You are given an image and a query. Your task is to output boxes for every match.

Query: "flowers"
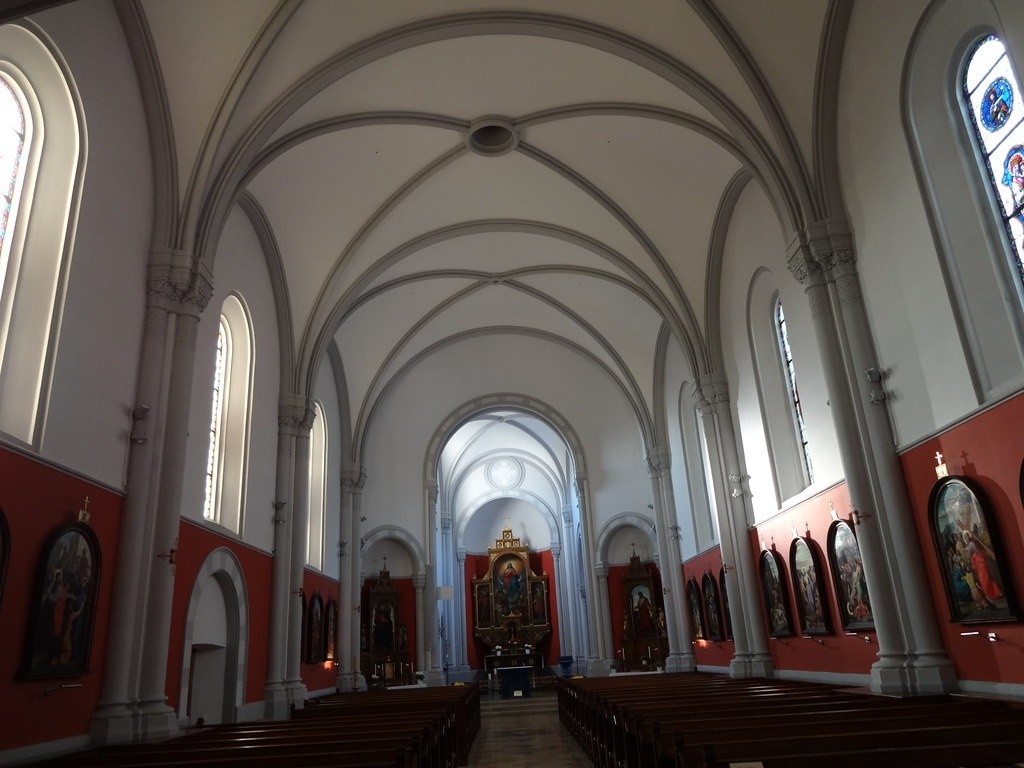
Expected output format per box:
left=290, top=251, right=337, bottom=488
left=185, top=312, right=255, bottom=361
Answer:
left=495, top=646, right=502, bottom=650
left=506, top=637, right=523, bottom=648
left=525, top=644, right=532, bottom=649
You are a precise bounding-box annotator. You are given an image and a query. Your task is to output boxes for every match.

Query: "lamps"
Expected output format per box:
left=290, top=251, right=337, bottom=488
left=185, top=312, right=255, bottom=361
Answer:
left=438, top=586, right=455, bottom=668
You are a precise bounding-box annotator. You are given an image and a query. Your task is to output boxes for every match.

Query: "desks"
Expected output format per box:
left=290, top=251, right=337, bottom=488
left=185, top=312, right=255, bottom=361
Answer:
left=484, top=651, right=545, bottom=696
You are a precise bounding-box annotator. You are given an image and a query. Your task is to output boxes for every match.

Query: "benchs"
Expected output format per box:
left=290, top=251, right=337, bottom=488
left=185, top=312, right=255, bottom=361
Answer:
left=555, top=669, right=1024, bottom=768
left=18, top=684, right=482, bottom=768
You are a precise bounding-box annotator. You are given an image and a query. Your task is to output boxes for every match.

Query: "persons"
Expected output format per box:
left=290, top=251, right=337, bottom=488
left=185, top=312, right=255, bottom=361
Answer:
left=622, top=609, right=630, bottom=633
left=399, top=624, right=408, bottom=650
left=361, top=622, right=369, bottom=652
left=656, top=607, right=667, bottom=637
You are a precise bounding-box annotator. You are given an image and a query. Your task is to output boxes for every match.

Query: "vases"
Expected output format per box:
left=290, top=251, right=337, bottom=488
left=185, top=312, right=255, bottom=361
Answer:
left=525, top=649, right=530, bottom=654
left=496, top=651, right=501, bottom=656
left=509, top=646, right=518, bottom=655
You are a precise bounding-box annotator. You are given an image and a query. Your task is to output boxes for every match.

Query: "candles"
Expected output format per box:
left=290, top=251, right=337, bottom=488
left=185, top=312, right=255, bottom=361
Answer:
left=374, top=664, right=377, bottom=676
left=411, top=662, right=413, bottom=675
left=648, top=647, right=651, bottom=659
left=401, top=662, right=402, bottom=674
left=622, top=648, right=625, bottom=660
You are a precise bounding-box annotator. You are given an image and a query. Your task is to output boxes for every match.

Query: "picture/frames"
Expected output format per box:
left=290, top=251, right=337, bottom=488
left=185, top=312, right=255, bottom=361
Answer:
left=686, top=567, right=734, bottom=642
left=16, top=522, right=103, bottom=682
left=826, top=519, right=875, bottom=631
left=927, top=475, right=1024, bottom=626
left=788, top=536, right=831, bottom=634
left=300, top=592, right=337, bottom=664
left=758, top=550, right=793, bottom=638
